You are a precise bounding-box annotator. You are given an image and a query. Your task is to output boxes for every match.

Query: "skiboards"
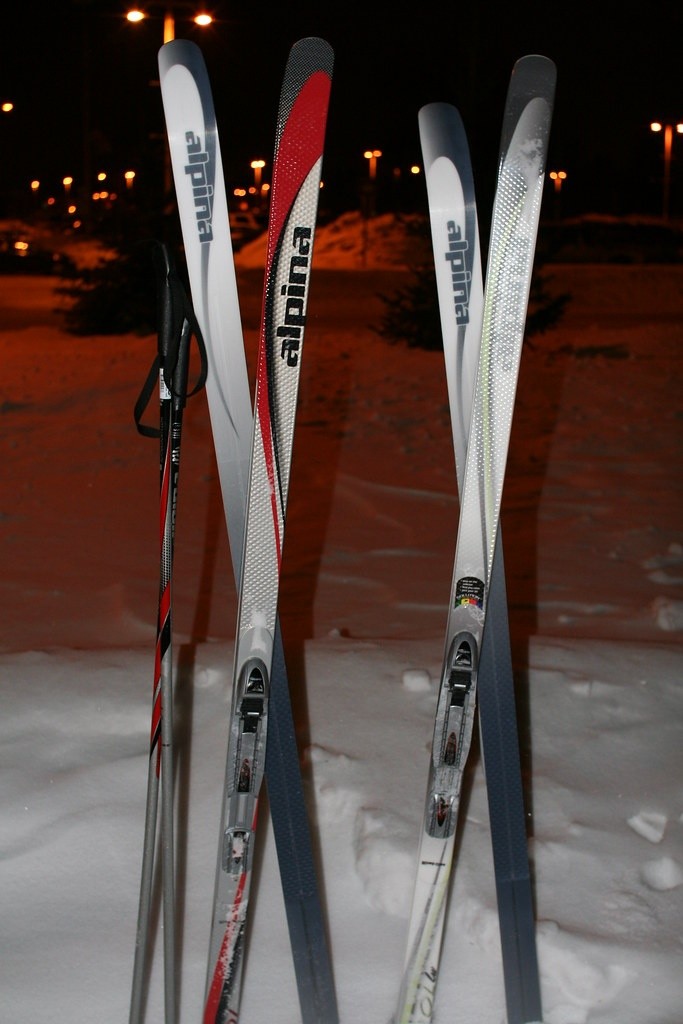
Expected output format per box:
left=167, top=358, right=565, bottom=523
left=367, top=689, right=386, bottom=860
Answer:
left=90, top=0, right=364, bottom=1024
left=387, top=0, right=585, bottom=1024
left=238, top=707, right=242, bottom=714
